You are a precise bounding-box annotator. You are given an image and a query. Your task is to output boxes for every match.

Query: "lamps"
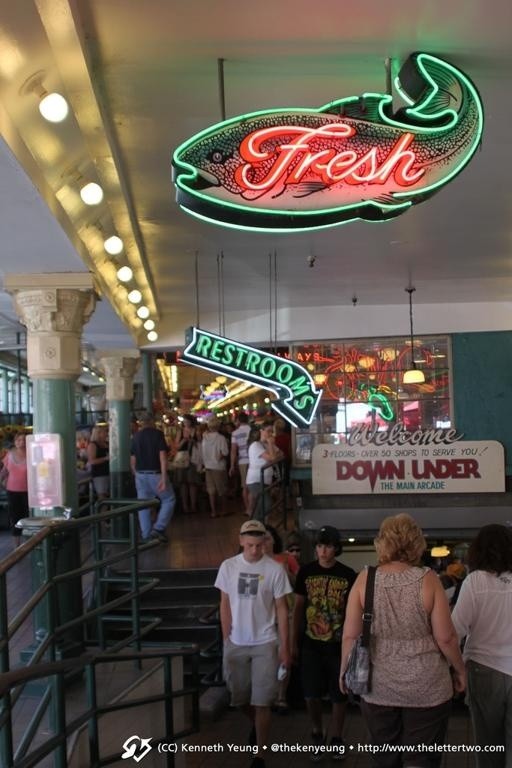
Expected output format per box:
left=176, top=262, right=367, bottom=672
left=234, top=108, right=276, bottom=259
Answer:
left=18, top=70, right=69, bottom=124
left=90, top=214, right=124, bottom=256
left=104, top=254, right=133, bottom=282
left=61, top=159, right=105, bottom=206
left=402, top=287, right=426, bottom=384
left=136, top=305, right=159, bottom=343
left=117, top=281, right=142, bottom=304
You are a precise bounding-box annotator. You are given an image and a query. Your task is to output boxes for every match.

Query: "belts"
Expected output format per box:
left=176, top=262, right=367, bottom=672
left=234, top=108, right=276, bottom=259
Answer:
left=135, top=470, right=161, bottom=475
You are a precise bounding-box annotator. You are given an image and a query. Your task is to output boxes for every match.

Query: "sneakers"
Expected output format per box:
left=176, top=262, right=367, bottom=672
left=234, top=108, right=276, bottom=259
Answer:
left=209, top=510, right=250, bottom=518
left=308, top=730, right=347, bottom=761
left=150, top=529, right=171, bottom=543
left=241, top=723, right=270, bottom=767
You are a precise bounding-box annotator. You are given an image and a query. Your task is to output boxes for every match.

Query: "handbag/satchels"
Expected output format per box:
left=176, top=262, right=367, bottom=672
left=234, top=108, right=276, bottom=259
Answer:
left=342, top=563, right=378, bottom=696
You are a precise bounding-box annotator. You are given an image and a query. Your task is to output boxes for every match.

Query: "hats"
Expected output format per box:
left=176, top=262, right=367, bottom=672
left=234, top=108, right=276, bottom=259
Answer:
left=312, top=525, right=344, bottom=558
left=239, top=519, right=267, bottom=535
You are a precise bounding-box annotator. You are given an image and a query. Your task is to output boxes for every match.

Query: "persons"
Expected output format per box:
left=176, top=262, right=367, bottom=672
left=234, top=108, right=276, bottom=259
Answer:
left=0, top=402, right=512, bottom=767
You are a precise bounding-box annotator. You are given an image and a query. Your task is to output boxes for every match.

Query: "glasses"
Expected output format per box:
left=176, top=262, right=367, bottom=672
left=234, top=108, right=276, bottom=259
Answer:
left=289, top=547, right=301, bottom=553
